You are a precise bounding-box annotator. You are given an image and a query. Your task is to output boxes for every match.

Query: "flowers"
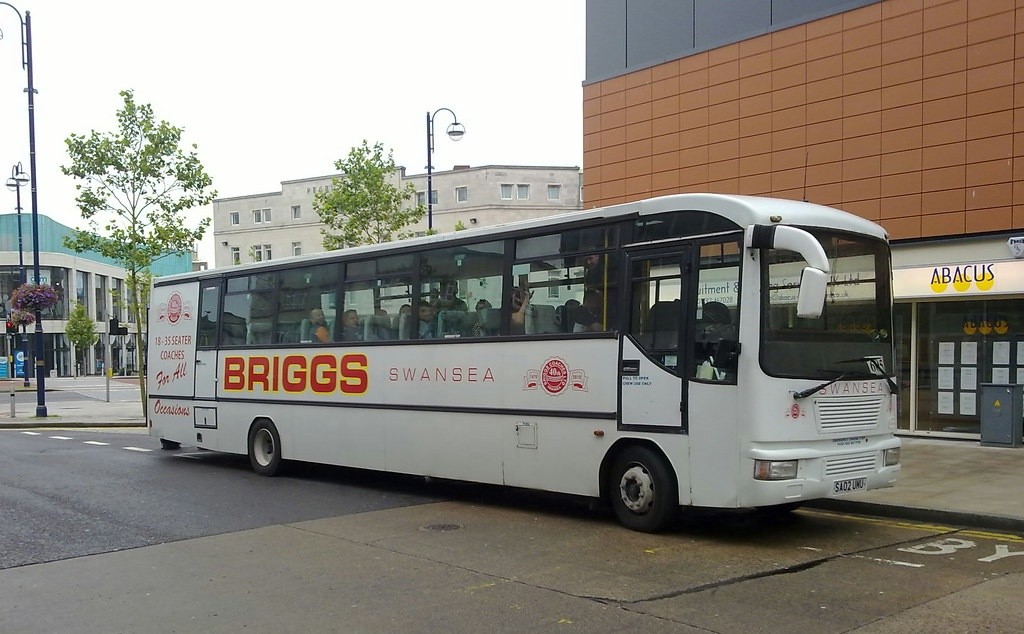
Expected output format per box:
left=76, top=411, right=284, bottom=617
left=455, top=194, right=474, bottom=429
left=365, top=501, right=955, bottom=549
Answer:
left=11, top=309, right=35, bottom=326
left=11, top=284, right=57, bottom=311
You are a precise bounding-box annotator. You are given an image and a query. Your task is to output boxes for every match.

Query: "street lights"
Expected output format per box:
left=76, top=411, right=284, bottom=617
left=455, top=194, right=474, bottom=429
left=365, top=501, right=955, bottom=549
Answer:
left=5, top=161, right=31, bottom=389
left=426, top=108, right=466, bottom=238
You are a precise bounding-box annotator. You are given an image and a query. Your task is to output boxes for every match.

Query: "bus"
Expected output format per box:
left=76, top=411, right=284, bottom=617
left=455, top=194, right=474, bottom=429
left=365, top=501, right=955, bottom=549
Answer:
left=144, top=192, right=901, bottom=534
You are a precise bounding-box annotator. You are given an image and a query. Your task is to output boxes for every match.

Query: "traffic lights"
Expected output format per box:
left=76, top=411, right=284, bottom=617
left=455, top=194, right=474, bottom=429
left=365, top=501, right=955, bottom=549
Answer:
left=6, top=321, right=12, bottom=334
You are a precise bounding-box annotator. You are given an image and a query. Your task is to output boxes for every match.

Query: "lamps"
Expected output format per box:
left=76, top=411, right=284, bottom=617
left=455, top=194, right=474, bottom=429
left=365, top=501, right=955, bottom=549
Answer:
left=222, top=242, right=227, bottom=246
left=470, top=218, right=476, bottom=224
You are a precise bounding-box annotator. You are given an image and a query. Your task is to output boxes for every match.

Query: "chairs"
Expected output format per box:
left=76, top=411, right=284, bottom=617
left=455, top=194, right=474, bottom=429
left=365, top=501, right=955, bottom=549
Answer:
left=221, top=302, right=732, bottom=347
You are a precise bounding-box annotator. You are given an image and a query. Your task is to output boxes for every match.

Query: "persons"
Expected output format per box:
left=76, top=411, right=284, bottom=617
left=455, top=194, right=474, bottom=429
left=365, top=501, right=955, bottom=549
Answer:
left=344, top=310, right=359, bottom=330
left=375, top=303, right=412, bottom=316
left=418, top=301, right=435, bottom=339
left=434, top=280, right=467, bottom=310
left=308, top=309, right=329, bottom=342
left=570, top=289, right=603, bottom=332
left=470, top=298, right=493, bottom=337
